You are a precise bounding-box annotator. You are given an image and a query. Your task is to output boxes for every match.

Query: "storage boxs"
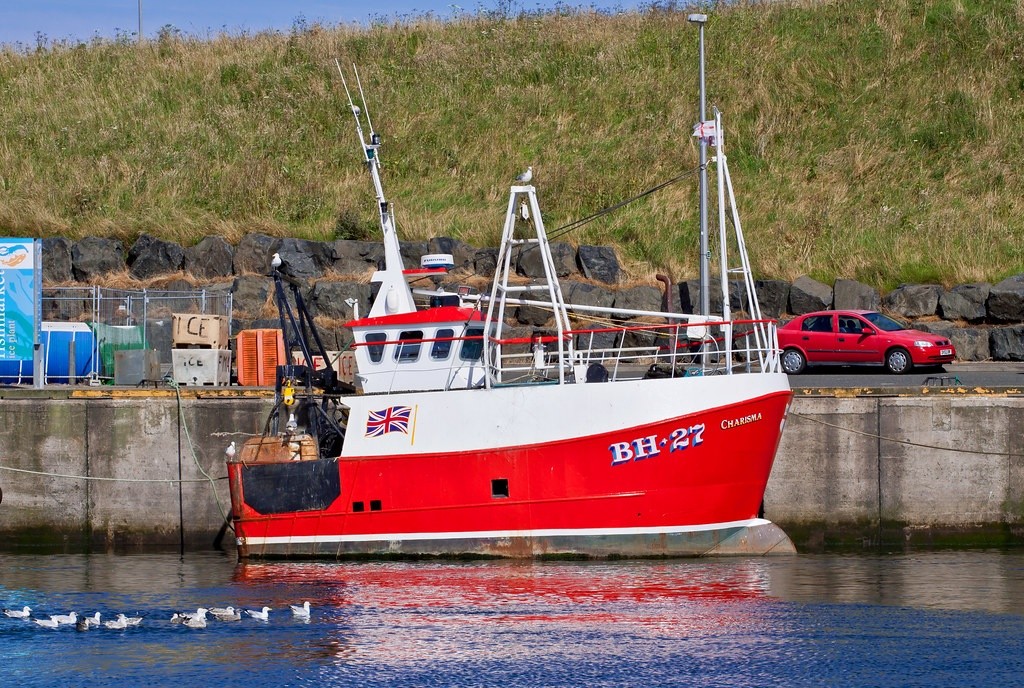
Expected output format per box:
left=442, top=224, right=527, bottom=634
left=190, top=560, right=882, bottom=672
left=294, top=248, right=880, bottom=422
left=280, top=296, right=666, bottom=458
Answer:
left=170, top=345, right=233, bottom=388
left=169, top=311, right=232, bottom=351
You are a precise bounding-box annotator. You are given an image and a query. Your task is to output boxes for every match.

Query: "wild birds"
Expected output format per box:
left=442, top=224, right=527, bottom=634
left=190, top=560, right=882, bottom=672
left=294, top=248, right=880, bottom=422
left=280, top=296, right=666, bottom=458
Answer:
left=0, top=605, right=33, bottom=618
left=76, top=617, right=90, bottom=632
left=289, top=601, right=312, bottom=616
left=31, top=616, right=59, bottom=627
left=101, top=617, right=127, bottom=629
left=244, top=607, right=272, bottom=619
left=86, top=611, right=101, bottom=629
left=208, top=606, right=235, bottom=616
left=170, top=608, right=208, bottom=628
left=115, top=613, right=143, bottom=625
left=214, top=609, right=244, bottom=621
left=48, top=612, right=79, bottom=624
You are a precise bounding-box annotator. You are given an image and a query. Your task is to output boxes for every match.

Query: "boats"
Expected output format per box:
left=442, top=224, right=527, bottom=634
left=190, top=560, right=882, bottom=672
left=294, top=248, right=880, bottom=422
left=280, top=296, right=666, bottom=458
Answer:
left=224, top=58, right=801, bottom=559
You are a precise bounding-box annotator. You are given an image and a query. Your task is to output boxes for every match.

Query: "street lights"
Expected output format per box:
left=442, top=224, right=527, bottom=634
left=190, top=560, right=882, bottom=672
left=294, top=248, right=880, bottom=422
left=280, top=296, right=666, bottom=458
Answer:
left=685, top=11, right=714, bottom=376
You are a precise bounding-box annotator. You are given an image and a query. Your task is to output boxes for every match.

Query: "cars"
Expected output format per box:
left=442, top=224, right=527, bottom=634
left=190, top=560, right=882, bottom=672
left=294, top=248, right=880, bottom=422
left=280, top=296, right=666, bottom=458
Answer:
left=774, top=309, right=957, bottom=375
